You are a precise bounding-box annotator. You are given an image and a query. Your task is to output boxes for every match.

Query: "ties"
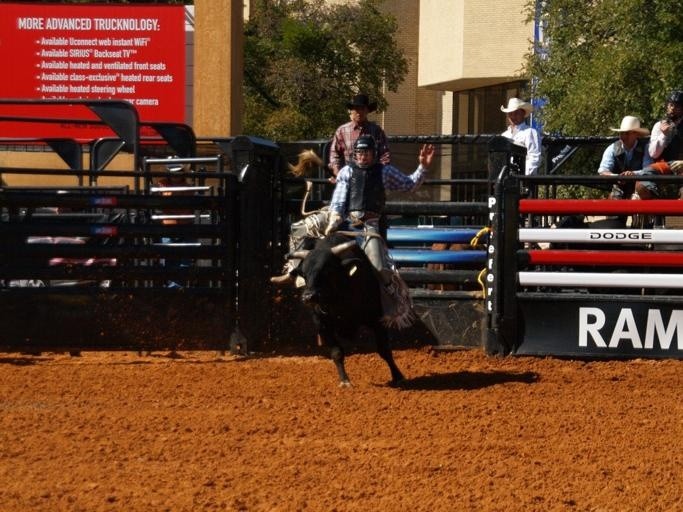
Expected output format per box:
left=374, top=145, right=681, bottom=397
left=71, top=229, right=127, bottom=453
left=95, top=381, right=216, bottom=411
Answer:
left=624, top=151, right=630, bottom=170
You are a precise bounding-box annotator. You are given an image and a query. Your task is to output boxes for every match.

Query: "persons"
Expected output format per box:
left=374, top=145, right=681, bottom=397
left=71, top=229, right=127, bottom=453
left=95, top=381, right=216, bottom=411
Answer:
left=1, top=153, right=204, bottom=290
left=597, top=114, right=654, bottom=232
left=327, top=94, right=392, bottom=183
left=270, top=133, right=436, bottom=303
left=647, top=89, right=683, bottom=230
left=498, top=97, right=542, bottom=250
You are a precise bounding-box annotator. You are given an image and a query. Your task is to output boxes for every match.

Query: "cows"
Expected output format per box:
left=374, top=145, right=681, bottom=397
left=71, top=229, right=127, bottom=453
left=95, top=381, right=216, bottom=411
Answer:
left=270, top=150, right=408, bottom=396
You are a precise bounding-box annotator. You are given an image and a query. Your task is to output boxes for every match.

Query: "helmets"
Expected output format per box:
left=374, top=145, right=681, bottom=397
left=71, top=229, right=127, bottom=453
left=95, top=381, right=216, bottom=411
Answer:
left=354, top=135, right=377, bottom=150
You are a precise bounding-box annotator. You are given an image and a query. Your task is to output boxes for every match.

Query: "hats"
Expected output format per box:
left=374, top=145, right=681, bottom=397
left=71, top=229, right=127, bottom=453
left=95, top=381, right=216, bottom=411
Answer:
left=500, top=98, right=533, bottom=117
left=344, top=94, right=377, bottom=112
left=610, top=116, right=649, bottom=136
left=667, top=92, right=682, bottom=106
